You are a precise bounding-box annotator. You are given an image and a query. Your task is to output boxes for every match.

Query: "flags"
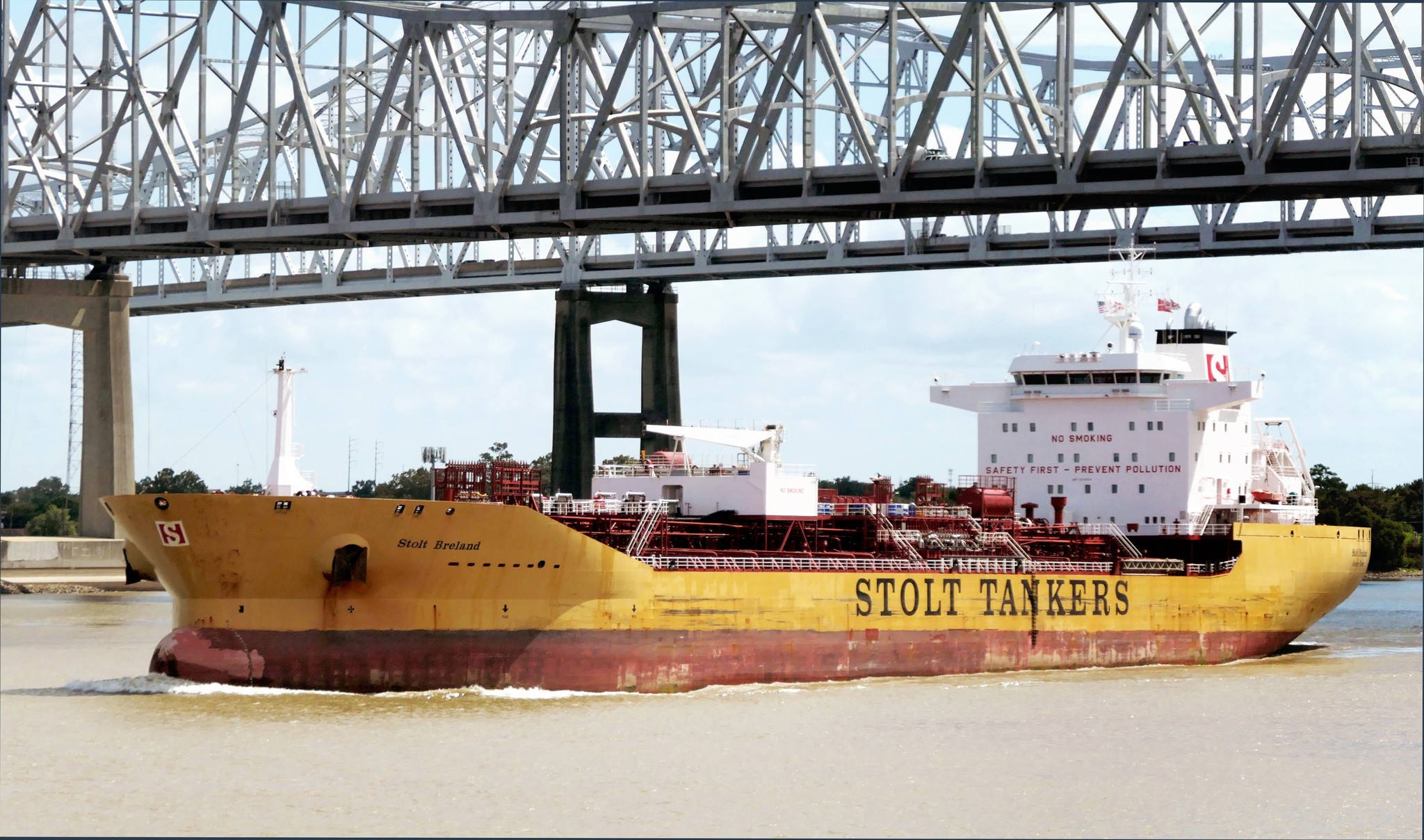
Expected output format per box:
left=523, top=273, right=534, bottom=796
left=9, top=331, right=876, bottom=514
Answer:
left=1158, top=297, right=1181, bottom=313
left=1097, top=300, right=1125, bottom=314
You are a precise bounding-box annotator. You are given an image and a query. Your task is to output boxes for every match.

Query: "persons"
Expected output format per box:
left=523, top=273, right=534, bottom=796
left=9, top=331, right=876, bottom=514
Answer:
left=1295, top=519, right=1301, bottom=524
left=593, top=494, right=603, bottom=510
left=621, top=495, right=625, bottom=508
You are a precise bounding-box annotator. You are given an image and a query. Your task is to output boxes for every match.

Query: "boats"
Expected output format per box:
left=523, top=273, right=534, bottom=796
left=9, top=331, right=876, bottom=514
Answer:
left=98, top=227, right=1373, bottom=692
